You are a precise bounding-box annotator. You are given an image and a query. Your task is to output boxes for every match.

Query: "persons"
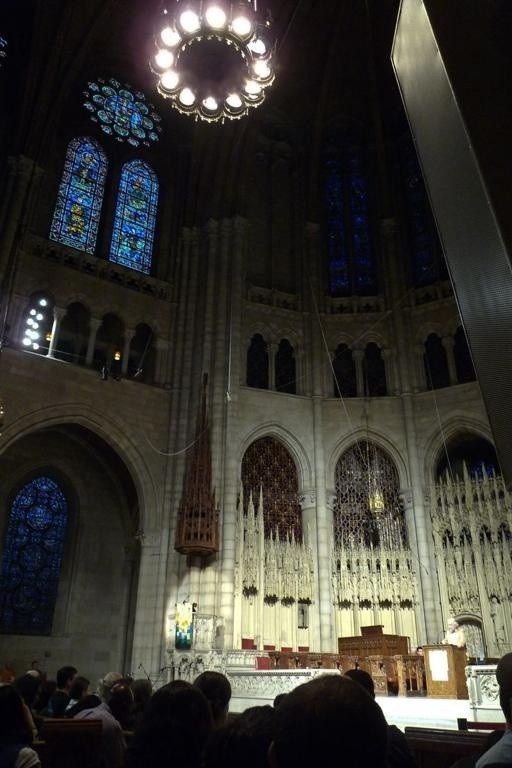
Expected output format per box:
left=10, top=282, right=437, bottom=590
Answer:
left=443, top=618, right=466, bottom=650
left=476, top=652, right=512, bottom=768
left=1, top=661, right=423, bottom=768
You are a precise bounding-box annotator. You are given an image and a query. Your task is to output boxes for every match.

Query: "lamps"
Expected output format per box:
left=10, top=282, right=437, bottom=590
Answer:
left=149, top=0, right=281, bottom=125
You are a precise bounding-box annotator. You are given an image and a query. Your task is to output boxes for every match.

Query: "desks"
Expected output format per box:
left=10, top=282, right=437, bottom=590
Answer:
left=465, top=664, right=501, bottom=710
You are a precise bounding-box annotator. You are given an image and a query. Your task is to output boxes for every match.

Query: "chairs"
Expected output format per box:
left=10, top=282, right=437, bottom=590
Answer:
left=405, top=725, right=494, bottom=768
left=241, top=639, right=309, bottom=652
left=257, top=657, right=272, bottom=669
left=43, top=718, right=104, bottom=767
